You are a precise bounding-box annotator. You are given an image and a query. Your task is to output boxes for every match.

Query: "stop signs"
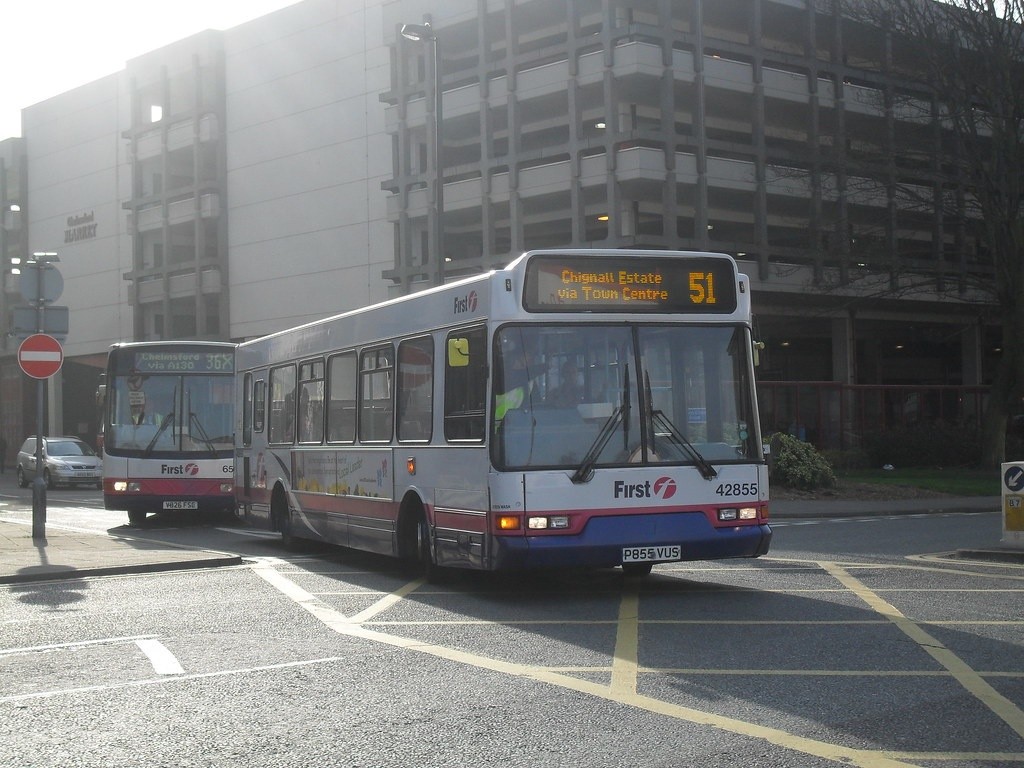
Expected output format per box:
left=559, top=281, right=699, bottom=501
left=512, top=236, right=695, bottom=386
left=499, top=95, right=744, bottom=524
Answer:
left=17, top=333, right=64, bottom=380
left=396, top=346, right=432, bottom=387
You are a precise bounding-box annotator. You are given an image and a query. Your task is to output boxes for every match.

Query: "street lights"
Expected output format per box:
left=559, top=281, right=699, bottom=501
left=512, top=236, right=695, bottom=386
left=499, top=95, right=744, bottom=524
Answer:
left=399, top=24, right=443, bottom=287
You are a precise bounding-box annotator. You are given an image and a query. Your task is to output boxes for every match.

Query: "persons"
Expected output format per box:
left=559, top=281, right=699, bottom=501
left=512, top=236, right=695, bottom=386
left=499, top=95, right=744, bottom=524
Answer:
left=552, top=361, right=583, bottom=405
left=131, top=399, right=164, bottom=426
left=281, top=387, right=309, bottom=436
left=496, top=351, right=552, bottom=427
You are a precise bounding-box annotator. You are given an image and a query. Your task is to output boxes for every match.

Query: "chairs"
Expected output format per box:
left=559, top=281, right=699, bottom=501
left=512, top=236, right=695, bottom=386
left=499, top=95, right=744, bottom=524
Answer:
left=275, top=406, right=431, bottom=442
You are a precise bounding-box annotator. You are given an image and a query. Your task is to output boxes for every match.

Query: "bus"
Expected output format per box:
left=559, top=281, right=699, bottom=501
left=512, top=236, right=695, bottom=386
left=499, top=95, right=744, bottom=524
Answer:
left=233, top=248, right=773, bottom=583
left=94, top=340, right=238, bottom=527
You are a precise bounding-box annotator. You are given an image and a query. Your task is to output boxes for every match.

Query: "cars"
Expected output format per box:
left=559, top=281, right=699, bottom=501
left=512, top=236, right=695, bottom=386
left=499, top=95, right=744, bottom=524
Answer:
left=15, top=434, right=103, bottom=490
